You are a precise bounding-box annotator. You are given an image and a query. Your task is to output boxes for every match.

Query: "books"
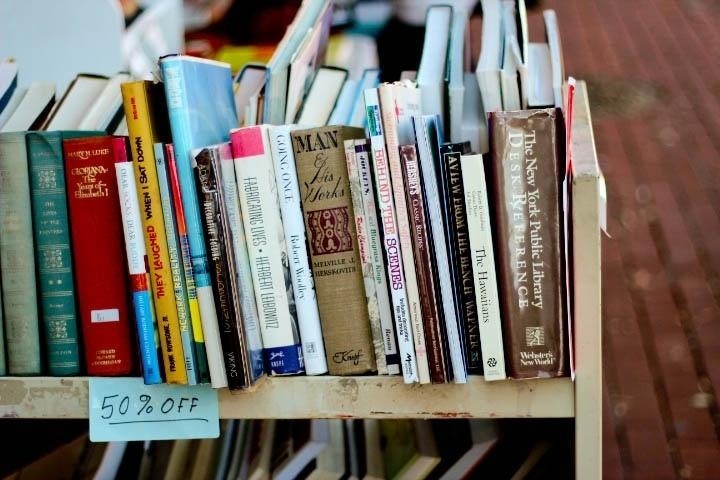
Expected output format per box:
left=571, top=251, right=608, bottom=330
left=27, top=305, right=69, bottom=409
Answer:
left=1, top=2, right=577, bottom=479
